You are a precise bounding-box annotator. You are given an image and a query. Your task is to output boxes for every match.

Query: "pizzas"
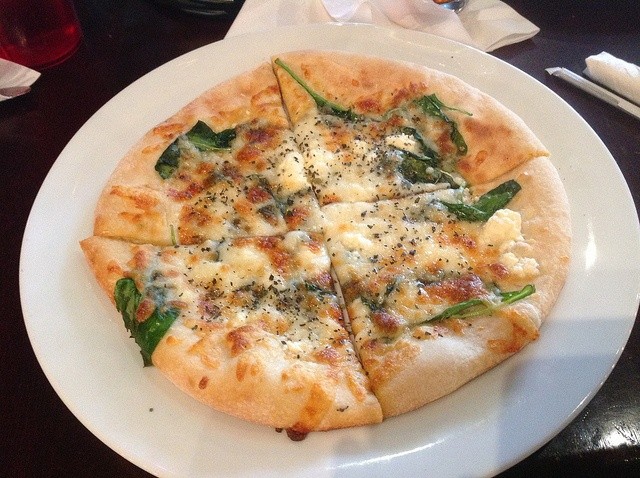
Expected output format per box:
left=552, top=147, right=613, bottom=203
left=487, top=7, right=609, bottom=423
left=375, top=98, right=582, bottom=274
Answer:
left=79, top=48, right=572, bottom=433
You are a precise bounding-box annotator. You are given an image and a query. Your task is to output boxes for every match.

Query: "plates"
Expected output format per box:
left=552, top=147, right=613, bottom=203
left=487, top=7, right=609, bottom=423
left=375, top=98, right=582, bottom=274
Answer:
left=18, top=21, right=639, bottom=478
